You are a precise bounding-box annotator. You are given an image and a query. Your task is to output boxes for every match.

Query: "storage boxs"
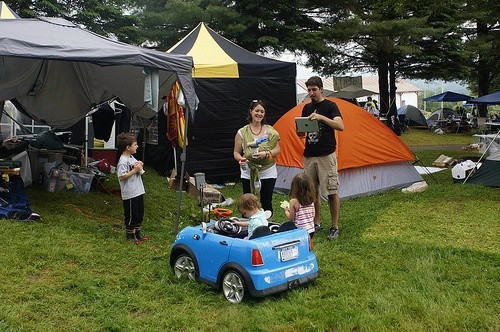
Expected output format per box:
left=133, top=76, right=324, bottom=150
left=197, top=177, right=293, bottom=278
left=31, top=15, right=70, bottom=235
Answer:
left=43, top=164, right=96, bottom=194
left=170, top=168, right=221, bottom=203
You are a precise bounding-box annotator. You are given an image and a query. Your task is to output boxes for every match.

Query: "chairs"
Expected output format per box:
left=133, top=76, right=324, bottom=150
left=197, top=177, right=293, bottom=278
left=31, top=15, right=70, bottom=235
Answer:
left=276, top=221, right=297, bottom=232
left=249, top=226, right=271, bottom=240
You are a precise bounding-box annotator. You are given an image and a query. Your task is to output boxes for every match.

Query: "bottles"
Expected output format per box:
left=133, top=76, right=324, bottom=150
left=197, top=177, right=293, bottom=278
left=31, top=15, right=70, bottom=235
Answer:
left=240, top=158, right=247, bottom=173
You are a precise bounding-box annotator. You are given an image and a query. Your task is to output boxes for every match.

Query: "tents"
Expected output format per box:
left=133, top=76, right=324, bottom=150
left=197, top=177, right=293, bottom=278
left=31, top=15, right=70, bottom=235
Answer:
left=397, top=92, right=500, bottom=134
left=103, top=22, right=299, bottom=188
left=273, top=96, right=440, bottom=203
left=0, top=16, right=196, bottom=236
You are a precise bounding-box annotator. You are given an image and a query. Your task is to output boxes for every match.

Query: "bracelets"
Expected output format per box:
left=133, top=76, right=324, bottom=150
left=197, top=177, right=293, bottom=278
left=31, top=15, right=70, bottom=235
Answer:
left=265, top=150, right=271, bottom=158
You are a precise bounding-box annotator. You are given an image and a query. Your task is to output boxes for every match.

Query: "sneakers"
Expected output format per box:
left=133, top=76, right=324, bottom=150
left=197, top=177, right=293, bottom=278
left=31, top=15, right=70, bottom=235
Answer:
left=315, top=224, right=325, bottom=233
left=327, top=226, right=339, bottom=240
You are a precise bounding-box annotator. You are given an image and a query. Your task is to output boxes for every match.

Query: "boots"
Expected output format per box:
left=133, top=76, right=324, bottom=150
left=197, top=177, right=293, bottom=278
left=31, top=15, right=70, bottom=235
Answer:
left=134, top=225, right=149, bottom=240
left=125, top=229, right=141, bottom=245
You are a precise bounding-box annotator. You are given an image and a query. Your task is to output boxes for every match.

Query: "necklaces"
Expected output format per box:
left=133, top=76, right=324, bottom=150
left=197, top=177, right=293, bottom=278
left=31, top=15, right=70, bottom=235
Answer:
left=249, top=124, right=262, bottom=135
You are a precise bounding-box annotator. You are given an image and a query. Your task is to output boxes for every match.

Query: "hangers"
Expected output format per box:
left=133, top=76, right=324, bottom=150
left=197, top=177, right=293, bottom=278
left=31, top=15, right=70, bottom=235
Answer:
left=114, top=108, right=122, bottom=115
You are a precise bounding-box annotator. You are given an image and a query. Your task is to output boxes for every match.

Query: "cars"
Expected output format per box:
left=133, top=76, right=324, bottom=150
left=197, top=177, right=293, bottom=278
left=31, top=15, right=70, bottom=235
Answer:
left=169, top=208, right=320, bottom=304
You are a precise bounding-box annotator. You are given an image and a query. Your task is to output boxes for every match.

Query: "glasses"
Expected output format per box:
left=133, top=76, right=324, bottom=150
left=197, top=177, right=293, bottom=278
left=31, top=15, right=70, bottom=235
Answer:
left=251, top=100, right=265, bottom=105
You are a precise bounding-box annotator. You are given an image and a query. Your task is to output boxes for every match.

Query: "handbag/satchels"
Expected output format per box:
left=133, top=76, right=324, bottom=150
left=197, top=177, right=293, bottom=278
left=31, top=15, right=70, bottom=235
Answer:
left=177, top=89, right=186, bottom=108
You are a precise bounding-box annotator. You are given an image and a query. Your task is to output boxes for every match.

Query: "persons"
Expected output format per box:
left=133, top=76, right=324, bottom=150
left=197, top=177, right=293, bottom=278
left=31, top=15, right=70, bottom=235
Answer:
left=233, top=100, right=281, bottom=221
left=116, top=132, right=150, bottom=245
left=283, top=173, right=316, bottom=238
left=230, top=192, right=272, bottom=240
left=296, top=76, right=344, bottom=240
left=364, top=98, right=380, bottom=117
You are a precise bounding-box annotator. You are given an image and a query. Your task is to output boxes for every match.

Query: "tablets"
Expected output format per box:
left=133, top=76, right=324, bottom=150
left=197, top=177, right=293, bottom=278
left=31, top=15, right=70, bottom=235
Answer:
left=295, top=117, right=319, bottom=132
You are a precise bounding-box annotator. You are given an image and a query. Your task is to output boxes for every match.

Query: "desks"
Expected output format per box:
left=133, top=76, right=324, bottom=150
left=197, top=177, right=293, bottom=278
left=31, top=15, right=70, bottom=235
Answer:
left=70, top=144, right=118, bottom=166
